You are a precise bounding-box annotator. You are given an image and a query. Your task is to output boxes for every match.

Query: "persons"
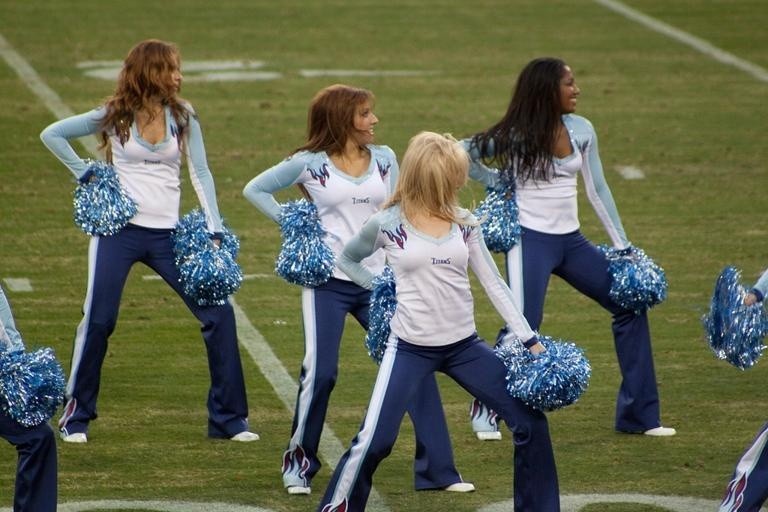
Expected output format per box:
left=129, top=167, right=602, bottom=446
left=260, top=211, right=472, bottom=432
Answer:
left=716, top=267, right=768, bottom=511
left=315, top=129, right=561, bottom=510
left=0, top=282, right=58, bottom=511
left=455, top=58, right=676, bottom=442
left=38, top=38, right=261, bottom=442
left=242, top=85, right=478, bottom=497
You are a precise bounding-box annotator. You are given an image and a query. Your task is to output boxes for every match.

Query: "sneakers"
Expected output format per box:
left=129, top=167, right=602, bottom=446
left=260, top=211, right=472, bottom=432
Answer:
left=231, top=433, right=261, bottom=442
left=643, top=426, right=677, bottom=436
left=288, top=484, right=311, bottom=495
left=477, top=432, right=502, bottom=439
left=64, top=433, right=88, bottom=443
left=443, top=483, right=474, bottom=492
